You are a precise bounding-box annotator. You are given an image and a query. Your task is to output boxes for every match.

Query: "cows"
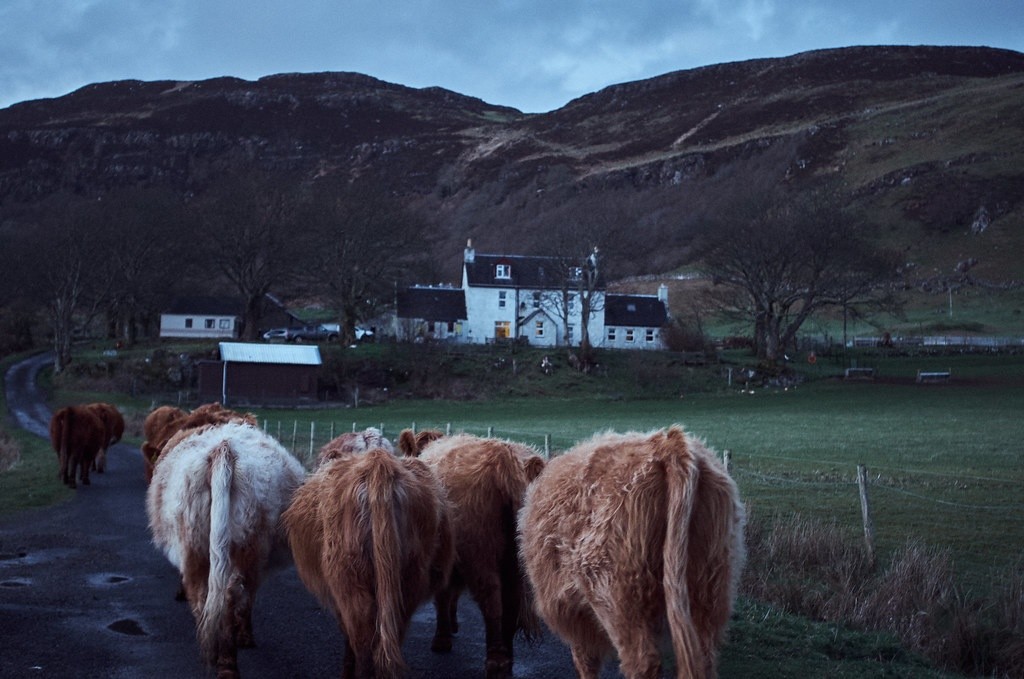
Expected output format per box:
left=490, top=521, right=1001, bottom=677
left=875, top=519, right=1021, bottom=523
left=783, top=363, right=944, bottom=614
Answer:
left=50, top=402, right=127, bottom=489
left=140, top=401, right=749, bottom=678
